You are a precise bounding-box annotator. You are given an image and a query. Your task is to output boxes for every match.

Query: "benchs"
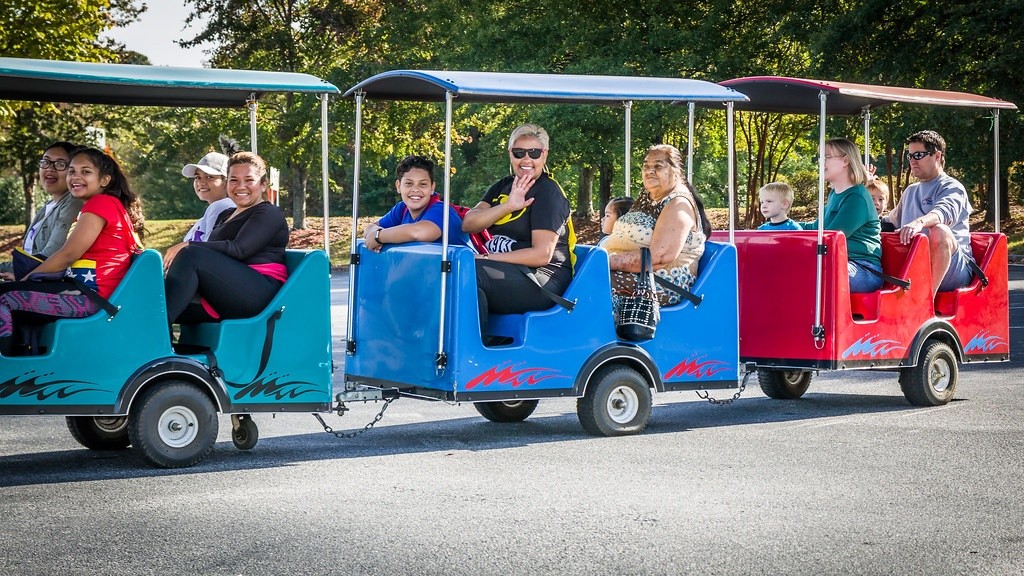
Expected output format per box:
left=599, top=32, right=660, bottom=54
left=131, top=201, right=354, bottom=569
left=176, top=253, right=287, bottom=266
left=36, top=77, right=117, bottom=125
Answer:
left=26, top=229, right=1007, bottom=416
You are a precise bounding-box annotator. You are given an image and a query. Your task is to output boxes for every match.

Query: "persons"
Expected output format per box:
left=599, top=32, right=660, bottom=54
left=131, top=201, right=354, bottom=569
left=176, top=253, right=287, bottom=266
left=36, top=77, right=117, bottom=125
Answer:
left=363, top=155, right=482, bottom=255
left=462, top=123, right=578, bottom=346
left=179, top=152, right=238, bottom=246
left=865, top=178, right=898, bottom=233
left=597, top=144, right=712, bottom=308
left=756, top=181, right=804, bottom=230
left=0, top=141, right=88, bottom=282
left=601, top=196, right=635, bottom=235
left=163, top=151, right=289, bottom=352
left=879, top=130, right=973, bottom=301
left=0, top=149, right=146, bottom=359
left=795, top=138, right=884, bottom=293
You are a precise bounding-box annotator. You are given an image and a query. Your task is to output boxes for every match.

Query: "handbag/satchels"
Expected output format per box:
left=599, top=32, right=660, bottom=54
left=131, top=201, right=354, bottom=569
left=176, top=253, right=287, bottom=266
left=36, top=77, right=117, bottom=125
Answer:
left=616, top=248, right=657, bottom=341
left=12, top=246, right=72, bottom=281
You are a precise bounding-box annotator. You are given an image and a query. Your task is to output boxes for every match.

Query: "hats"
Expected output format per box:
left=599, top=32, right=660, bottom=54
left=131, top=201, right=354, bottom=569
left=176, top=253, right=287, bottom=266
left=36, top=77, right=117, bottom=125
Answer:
left=182, top=152, right=229, bottom=178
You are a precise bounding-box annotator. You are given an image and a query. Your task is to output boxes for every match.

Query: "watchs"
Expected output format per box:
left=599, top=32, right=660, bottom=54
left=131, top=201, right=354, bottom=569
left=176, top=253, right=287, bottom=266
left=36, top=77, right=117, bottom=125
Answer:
left=375, top=228, right=383, bottom=245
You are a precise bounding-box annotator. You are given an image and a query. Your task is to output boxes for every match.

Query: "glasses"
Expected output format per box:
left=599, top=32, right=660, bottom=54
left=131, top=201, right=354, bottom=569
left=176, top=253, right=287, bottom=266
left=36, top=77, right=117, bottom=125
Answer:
left=36, top=159, right=71, bottom=171
left=907, top=151, right=943, bottom=161
left=510, top=148, right=544, bottom=159
left=817, top=155, right=844, bottom=161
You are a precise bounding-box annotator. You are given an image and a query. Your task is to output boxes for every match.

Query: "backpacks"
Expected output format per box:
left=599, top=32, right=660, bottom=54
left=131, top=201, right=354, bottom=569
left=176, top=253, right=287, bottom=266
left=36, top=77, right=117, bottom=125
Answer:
left=402, top=193, right=492, bottom=257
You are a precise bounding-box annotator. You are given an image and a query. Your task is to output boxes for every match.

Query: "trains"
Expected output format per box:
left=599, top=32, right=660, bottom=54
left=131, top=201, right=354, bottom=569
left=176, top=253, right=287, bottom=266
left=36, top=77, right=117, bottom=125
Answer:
left=0, top=54, right=1018, bottom=468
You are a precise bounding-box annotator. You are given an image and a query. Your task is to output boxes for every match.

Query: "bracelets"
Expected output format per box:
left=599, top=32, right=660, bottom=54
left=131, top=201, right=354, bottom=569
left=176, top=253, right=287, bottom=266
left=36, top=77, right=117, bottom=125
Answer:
left=483, top=253, right=488, bottom=260
